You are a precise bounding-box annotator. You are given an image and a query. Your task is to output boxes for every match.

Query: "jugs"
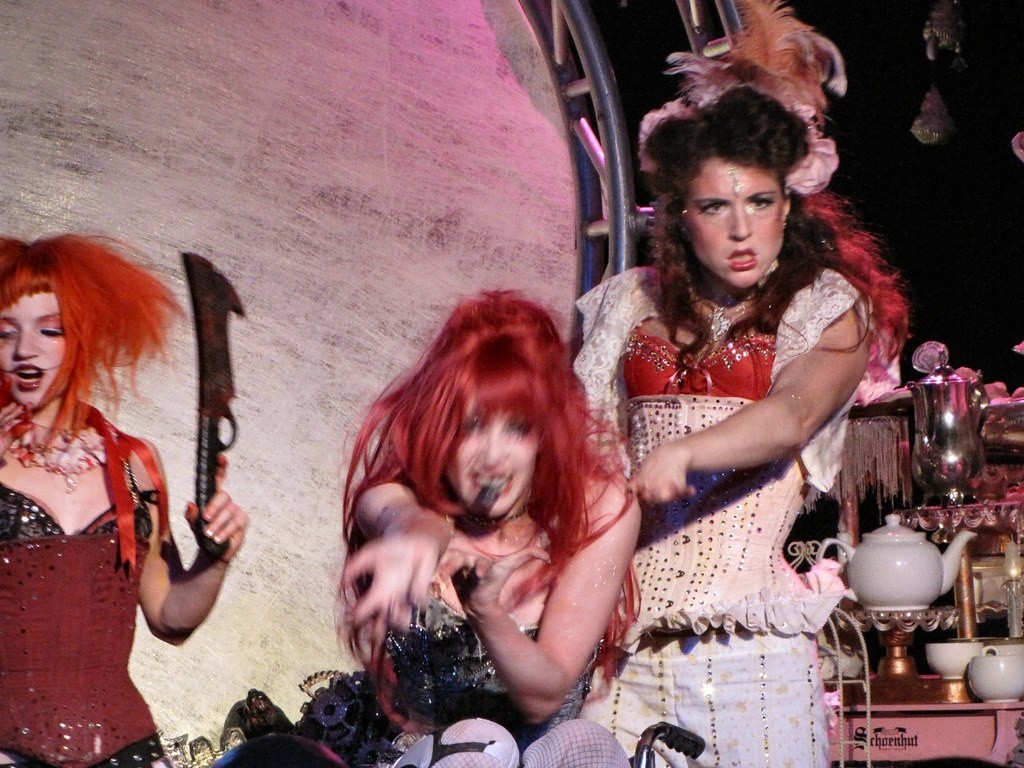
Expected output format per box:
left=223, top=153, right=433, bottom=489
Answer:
left=907, top=365, right=982, bottom=507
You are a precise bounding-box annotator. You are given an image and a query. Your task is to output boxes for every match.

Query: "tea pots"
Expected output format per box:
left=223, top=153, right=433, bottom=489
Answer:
left=817, top=514, right=978, bottom=611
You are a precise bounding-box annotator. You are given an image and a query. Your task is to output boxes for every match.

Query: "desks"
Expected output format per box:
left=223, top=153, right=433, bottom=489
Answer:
left=827, top=702, right=1024, bottom=768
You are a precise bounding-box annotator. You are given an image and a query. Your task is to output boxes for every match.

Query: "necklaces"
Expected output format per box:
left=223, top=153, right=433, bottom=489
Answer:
left=4, top=419, right=106, bottom=493
left=707, top=289, right=751, bottom=342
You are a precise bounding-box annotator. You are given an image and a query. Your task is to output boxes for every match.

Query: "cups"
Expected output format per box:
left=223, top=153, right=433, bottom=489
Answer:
left=988, top=644, right=1024, bottom=655
left=968, top=654, right=1024, bottom=703
left=925, top=641, right=999, bottom=678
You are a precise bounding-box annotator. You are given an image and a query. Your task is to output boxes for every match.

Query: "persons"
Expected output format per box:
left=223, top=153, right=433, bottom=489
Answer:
left=573, top=86, right=871, bottom=768
left=0, top=235, right=249, bottom=768
left=342, top=289, right=642, bottom=768
